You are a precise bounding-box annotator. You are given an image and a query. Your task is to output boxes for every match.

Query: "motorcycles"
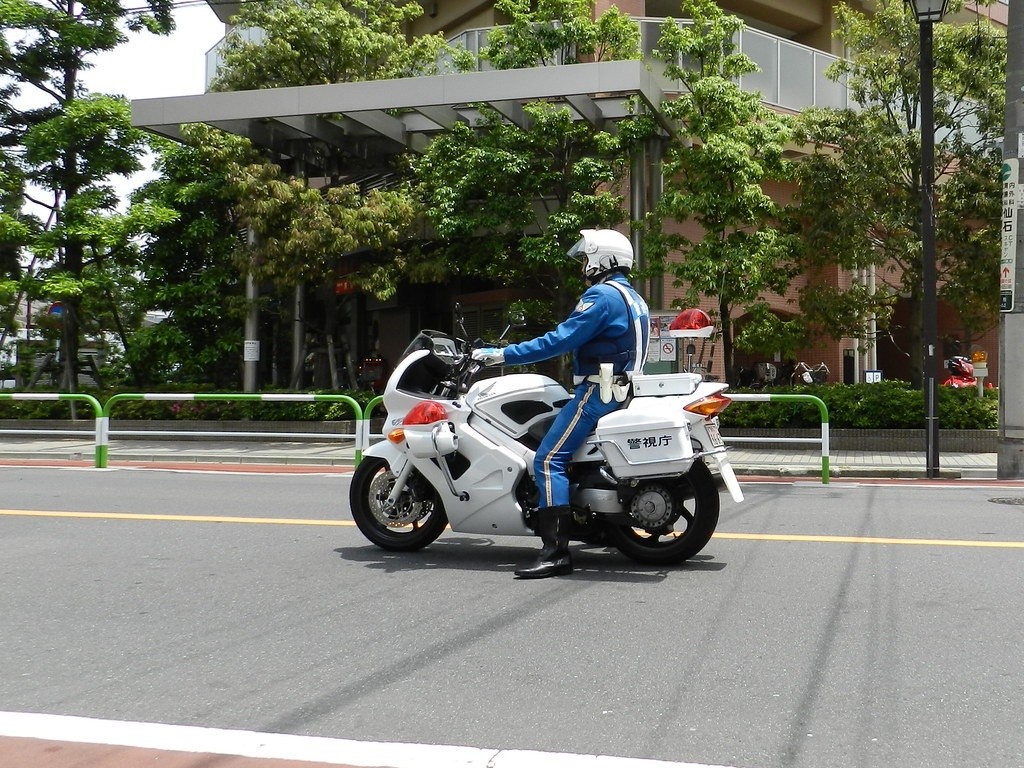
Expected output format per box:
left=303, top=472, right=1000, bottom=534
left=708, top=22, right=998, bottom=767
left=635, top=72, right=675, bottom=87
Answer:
left=347, top=302, right=745, bottom=568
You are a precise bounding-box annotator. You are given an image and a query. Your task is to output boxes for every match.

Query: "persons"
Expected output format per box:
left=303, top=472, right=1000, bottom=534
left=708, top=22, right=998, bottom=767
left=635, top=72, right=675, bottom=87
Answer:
left=469, top=229, right=653, bottom=577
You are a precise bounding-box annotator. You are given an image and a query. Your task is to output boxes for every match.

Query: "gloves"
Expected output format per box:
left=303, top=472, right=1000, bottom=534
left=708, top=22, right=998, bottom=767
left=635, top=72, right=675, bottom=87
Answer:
left=471, top=346, right=505, bottom=366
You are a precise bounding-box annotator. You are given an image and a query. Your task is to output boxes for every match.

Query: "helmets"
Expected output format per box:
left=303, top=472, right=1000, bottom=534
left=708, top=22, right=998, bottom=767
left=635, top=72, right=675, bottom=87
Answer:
left=579, top=229, right=633, bottom=279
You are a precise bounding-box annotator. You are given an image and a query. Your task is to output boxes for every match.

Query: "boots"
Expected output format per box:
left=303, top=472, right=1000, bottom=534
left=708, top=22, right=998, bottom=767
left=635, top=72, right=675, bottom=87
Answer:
left=515, top=507, right=573, bottom=577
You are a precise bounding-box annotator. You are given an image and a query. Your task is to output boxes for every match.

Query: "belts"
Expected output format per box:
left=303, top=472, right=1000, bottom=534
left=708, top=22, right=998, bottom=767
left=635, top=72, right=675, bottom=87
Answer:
left=571, top=373, right=628, bottom=386
